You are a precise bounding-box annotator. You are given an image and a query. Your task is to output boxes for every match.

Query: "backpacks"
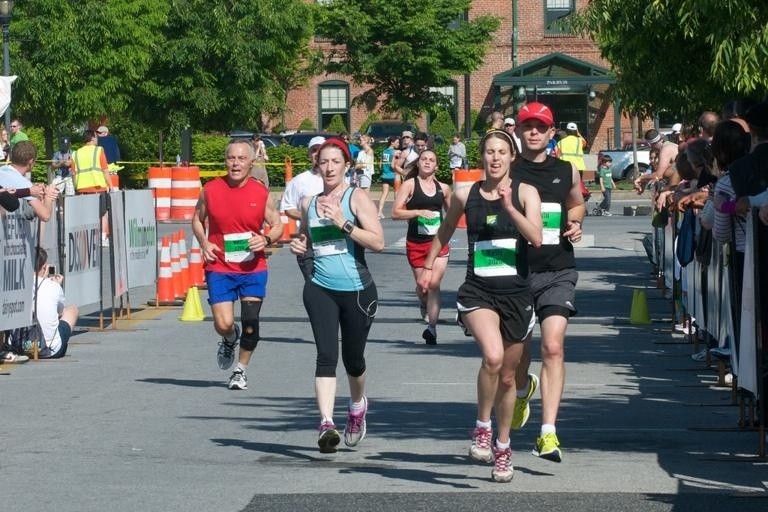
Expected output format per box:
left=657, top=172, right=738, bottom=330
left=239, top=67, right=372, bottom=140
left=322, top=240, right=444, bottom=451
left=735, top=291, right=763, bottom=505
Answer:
left=7, top=278, right=57, bottom=354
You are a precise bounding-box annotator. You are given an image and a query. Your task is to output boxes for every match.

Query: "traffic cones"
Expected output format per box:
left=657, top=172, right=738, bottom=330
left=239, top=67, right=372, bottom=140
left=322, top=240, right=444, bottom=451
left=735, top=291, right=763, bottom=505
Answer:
left=149, top=227, right=208, bottom=322
left=266, top=193, right=297, bottom=248
left=628, top=287, right=651, bottom=326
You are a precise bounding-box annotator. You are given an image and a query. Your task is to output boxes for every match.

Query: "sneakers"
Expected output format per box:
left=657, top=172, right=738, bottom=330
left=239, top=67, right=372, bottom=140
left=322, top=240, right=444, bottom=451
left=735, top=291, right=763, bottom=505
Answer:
left=318, top=421, right=341, bottom=452
left=674, top=323, right=733, bottom=384
left=101, top=238, right=109, bottom=246
left=228, top=372, right=247, bottom=389
left=216, top=324, right=240, bottom=370
left=342, top=394, right=368, bottom=447
left=420, top=305, right=562, bottom=483
left=0, top=351, right=29, bottom=363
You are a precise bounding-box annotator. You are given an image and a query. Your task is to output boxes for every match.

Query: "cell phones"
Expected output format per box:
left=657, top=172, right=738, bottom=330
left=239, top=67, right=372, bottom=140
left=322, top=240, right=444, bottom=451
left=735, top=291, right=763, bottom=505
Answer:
left=48, top=266, right=56, bottom=278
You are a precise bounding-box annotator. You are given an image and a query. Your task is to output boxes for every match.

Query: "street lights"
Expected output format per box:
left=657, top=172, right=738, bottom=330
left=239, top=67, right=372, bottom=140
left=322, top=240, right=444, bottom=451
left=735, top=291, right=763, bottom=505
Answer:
left=0, top=0, right=15, bottom=139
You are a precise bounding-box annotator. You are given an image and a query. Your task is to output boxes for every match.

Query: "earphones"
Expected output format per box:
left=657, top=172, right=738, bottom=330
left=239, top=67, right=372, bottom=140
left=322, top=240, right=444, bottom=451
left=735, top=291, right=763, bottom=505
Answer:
left=345, top=167, right=348, bottom=173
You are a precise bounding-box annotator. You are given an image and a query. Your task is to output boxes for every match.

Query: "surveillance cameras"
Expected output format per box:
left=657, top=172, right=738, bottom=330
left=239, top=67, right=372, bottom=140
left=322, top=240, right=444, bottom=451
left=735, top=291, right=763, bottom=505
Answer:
left=590, top=92, right=596, bottom=99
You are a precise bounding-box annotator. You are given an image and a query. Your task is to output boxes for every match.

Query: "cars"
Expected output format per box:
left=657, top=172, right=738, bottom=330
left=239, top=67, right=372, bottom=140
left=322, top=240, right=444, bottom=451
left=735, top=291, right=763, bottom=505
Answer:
left=621, top=139, right=649, bottom=150
left=363, top=121, right=417, bottom=144
left=230, top=128, right=350, bottom=150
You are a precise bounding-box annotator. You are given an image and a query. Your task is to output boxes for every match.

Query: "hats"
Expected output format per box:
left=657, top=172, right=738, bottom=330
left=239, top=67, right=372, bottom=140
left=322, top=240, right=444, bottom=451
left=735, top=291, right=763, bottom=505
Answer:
left=309, top=136, right=326, bottom=150
left=567, top=123, right=577, bottom=130
left=518, top=102, right=553, bottom=126
left=402, top=131, right=412, bottom=138
left=97, top=127, right=108, bottom=132
left=61, top=138, right=70, bottom=147
left=504, top=118, right=515, bottom=126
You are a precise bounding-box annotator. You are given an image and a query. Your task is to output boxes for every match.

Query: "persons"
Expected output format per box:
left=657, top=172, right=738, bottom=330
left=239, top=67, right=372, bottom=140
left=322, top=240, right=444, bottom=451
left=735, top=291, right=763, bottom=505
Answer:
left=507, top=102, right=586, bottom=464
left=486, top=113, right=522, bottom=154
left=282, top=136, right=327, bottom=283
left=546, top=123, right=587, bottom=180
left=417, top=129, right=543, bottom=488
left=391, top=149, right=455, bottom=346
left=339, top=131, right=466, bottom=220
left=597, top=154, right=616, bottom=216
left=0, top=119, right=119, bottom=363
left=252, top=134, right=267, bottom=159
left=191, top=138, right=284, bottom=391
left=263, top=235, right=272, bottom=246
left=288, top=138, right=385, bottom=454
left=632, top=111, right=768, bottom=365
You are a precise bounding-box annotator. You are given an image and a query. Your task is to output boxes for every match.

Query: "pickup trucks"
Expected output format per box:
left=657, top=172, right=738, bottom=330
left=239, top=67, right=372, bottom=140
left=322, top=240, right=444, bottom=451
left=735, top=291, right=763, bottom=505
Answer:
left=594, top=129, right=679, bottom=182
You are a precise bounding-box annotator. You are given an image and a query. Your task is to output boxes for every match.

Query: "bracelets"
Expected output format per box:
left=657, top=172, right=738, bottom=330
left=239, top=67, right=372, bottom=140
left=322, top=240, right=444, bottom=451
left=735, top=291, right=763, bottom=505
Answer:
left=341, top=219, right=355, bottom=235
left=422, top=266, right=433, bottom=272
left=571, top=217, right=580, bottom=228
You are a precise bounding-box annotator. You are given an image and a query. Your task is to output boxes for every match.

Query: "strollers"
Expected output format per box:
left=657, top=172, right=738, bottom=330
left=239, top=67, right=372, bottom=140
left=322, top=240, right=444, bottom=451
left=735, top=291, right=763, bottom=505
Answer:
left=579, top=180, right=600, bottom=216
